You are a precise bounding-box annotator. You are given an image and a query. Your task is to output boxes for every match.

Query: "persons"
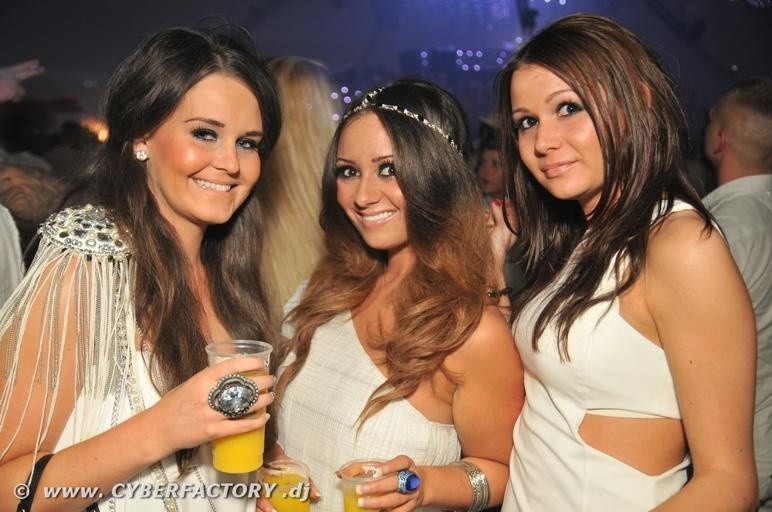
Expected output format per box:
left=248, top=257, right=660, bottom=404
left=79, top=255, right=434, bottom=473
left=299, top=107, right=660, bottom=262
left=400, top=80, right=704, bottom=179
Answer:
left=0, top=13, right=772, bottom=511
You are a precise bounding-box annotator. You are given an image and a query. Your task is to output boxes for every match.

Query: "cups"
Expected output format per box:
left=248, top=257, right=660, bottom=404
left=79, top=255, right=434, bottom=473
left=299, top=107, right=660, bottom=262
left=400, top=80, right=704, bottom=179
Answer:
left=340, top=460, right=394, bottom=512
left=269, top=463, right=310, bottom=512
left=205, top=339, right=273, bottom=473
